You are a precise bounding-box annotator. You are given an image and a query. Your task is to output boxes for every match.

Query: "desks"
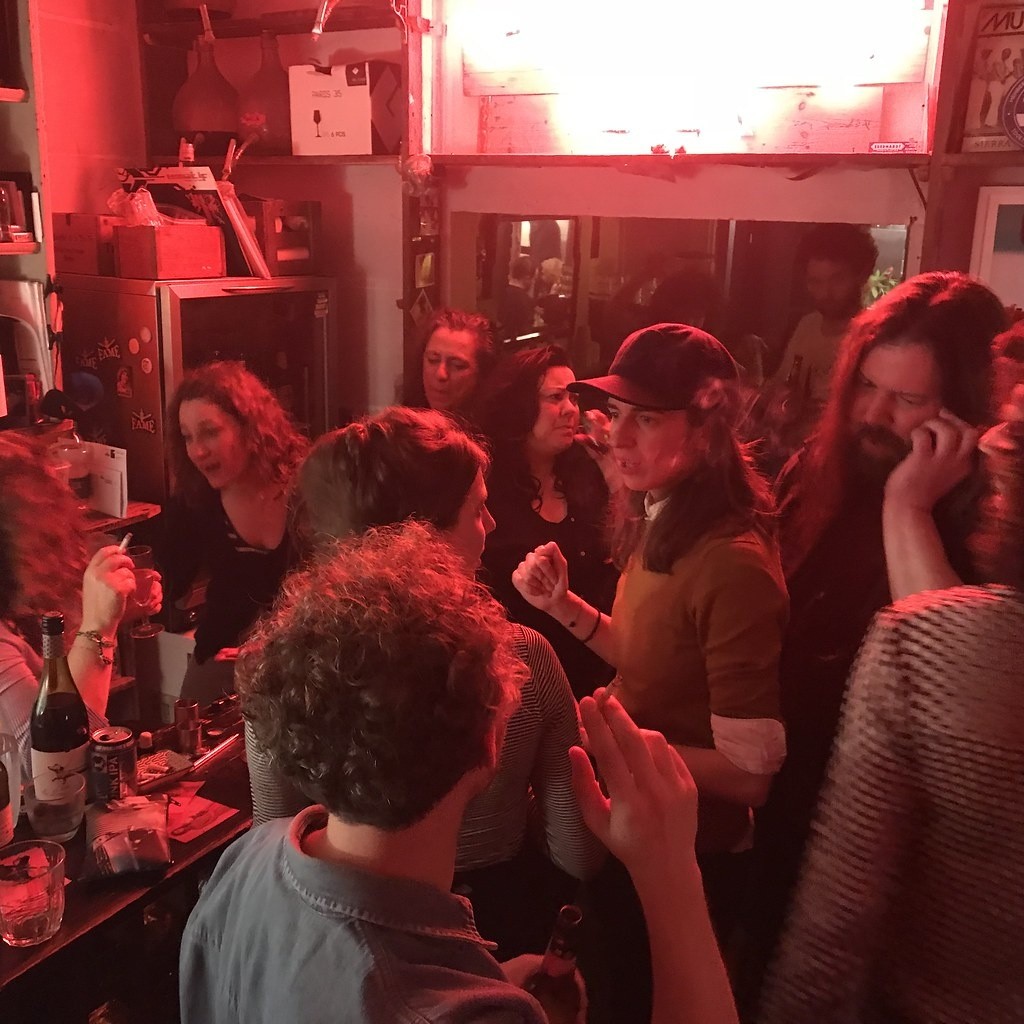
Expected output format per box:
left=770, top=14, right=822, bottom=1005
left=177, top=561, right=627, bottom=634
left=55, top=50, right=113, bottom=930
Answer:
left=1, top=654, right=248, bottom=1024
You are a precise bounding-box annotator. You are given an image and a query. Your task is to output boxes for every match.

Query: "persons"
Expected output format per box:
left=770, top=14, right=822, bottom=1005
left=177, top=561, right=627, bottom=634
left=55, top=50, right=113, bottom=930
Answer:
left=508, top=255, right=538, bottom=334
left=181, top=519, right=737, bottom=1023
left=300, top=407, right=496, bottom=585
left=512, top=322, right=790, bottom=963
left=759, top=226, right=1024, bottom=869
left=755, top=391, right=1024, bottom=1023
left=152, top=362, right=311, bottom=661
left=399, top=307, right=504, bottom=417
left=476, top=346, right=638, bottom=700
left=1, top=430, right=162, bottom=789
left=524, top=219, right=562, bottom=272
left=649, top=272, right=722, bottom=329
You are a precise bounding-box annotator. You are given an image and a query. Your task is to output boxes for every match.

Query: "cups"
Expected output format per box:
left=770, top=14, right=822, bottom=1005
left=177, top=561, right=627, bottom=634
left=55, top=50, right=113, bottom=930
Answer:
left=23, top=771, right=87, bottom=843
left=173, top=698, right=199, bottom=722
left=175, top=720, right=202, bottom=754
left=0, top=839, right=65, bottom=947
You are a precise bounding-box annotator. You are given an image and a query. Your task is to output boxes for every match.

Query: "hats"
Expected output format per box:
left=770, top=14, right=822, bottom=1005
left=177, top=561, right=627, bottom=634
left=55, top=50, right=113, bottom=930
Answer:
left=566, top=322, right=741, bottom=432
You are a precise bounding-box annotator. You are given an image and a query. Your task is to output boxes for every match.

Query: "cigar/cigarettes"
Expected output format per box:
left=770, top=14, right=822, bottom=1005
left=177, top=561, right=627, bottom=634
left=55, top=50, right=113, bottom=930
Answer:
left=119, top=534, right=132, bottom=550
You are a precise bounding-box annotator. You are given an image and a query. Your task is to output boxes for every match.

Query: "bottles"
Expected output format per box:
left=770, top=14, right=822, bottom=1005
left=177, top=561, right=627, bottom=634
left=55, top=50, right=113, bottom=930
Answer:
left=771, top=355, right=802, bottom=422
left=0, top=760, right=15, bottom=847
left=173, top=33, right=240, bottom=155
left=237, top=27, right=292, bottom=154
left=31, top=612, right=95, bottom=805
left=520, top=905, right=587, bottom=1024
left=60, top=418, right=93, bottom=513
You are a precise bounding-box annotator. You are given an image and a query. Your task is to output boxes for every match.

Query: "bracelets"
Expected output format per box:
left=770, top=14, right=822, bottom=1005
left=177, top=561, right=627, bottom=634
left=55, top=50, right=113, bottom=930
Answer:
left=75, top=630, right=117, bottom=664
left=562, top=596, right=584, bottom=628
left=579, top=606, right=601, bottom=643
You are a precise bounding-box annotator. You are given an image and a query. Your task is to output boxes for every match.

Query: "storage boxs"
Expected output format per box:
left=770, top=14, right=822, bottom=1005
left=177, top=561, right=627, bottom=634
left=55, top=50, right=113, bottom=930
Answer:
left=48, top=197, right=319, bottom=279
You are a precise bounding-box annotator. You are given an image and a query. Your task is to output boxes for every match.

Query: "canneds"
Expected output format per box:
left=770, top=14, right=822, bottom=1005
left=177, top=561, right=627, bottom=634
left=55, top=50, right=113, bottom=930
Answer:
left=88, top=727, right=137, bottom=807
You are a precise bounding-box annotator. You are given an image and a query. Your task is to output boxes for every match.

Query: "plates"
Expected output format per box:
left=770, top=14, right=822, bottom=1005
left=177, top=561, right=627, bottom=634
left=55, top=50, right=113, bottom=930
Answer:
left=136, top=749, right=194, bottom=792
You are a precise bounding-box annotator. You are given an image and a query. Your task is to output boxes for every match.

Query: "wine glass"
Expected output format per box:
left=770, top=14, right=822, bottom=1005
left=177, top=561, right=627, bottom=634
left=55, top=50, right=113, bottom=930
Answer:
left=121, top=545, right=164, bottom=638
